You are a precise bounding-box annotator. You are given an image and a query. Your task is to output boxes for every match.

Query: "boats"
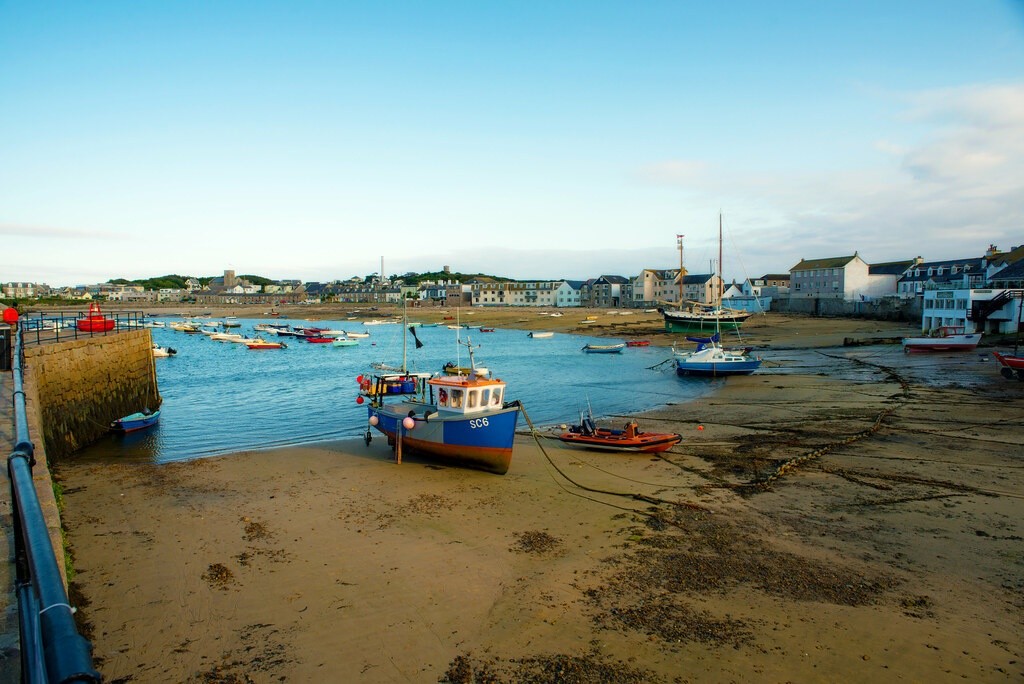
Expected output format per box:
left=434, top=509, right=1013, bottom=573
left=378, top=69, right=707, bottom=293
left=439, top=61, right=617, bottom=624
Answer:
left=526, top=331, right=554, bottom=338
left=537, top=308, right=658, bottom=323
left=558, top=415, right=683, bottom=455
left=112, top=408, right=163, bottom=434
left=581, top=342, right=627, bottom=353
left=346, top=305, right=530, bottom=332
left=153, top=343, right=178, bottom=357
left=903, top=326, right=984, bottom=355
left=993, top=352, right=1024, bottom=382
left=626, top=340, right=651, bottom=348
left=358, top=291, right=524, bottom=474
left=23, top=305, right=376, bottom=350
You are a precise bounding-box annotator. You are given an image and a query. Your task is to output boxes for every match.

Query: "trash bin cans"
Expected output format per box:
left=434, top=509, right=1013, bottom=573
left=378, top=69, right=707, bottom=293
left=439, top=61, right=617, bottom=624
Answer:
left=0, top=323, right=13, bottom=371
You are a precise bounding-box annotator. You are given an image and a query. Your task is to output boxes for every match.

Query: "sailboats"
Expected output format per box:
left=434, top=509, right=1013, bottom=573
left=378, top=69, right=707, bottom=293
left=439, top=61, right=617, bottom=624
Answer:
left=677, top=208, right=776, bottom=377
left=658, top=235, right=752, bottom=334
